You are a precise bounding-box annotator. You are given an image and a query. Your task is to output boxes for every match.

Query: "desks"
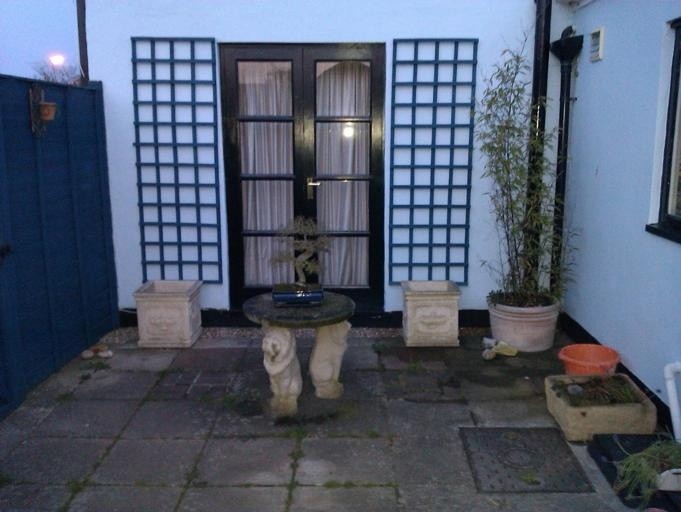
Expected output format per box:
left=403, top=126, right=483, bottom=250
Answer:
left=243, top=292, right=358, bottom=416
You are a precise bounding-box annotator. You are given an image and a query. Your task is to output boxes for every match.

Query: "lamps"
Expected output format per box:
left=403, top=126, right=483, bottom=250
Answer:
left=29, top=88, right=58, bottom=138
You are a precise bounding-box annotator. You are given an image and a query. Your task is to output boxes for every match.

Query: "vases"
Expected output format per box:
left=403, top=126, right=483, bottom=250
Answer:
left=559, top=343, right=622, bottom=377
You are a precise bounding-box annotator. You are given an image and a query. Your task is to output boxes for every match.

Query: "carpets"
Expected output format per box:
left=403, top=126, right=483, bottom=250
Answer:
left=459, top=427, right=597, bottom=494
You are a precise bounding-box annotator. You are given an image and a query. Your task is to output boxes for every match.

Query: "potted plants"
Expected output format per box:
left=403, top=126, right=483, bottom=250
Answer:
left=268, top=216, right=333, bottom=308
left=471, top=25, right=584, bottom=352
left=544, top=372, right=681, bottom=511
